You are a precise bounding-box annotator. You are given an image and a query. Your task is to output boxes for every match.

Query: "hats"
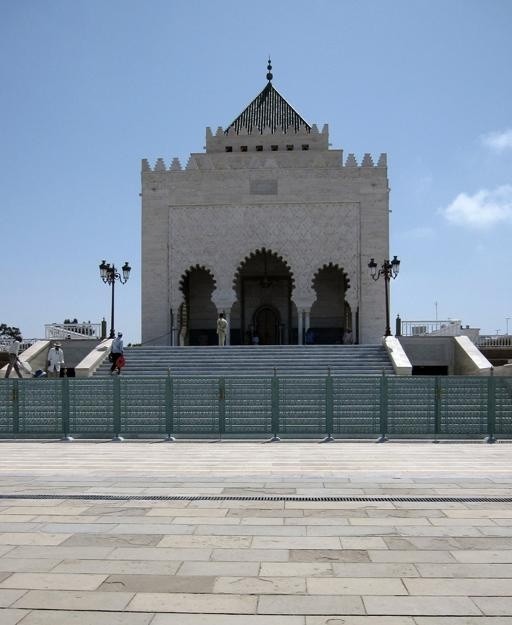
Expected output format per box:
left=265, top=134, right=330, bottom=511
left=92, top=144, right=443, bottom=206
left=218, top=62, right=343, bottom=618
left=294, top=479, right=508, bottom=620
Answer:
left=118, top=332, right=122, bottom=336
left=54, top=342, right=62, bottom=347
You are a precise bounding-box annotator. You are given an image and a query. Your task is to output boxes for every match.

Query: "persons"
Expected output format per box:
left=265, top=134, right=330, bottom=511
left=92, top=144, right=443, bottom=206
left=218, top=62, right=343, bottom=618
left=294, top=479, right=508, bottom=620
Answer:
left=47, top=341, right=65, bottom=377
left=216, top=313, right=228, bottom=346
left=6, top=336, right=23, bottom=379
left=110, top=331, right=124, bottom=375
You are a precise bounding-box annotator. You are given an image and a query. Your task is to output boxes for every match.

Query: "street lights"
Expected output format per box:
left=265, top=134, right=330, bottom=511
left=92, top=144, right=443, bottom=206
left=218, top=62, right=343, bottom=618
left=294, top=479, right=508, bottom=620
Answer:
left=98, top=258, right=131, bottom=342
left=367, top=256, right=400, bottom=338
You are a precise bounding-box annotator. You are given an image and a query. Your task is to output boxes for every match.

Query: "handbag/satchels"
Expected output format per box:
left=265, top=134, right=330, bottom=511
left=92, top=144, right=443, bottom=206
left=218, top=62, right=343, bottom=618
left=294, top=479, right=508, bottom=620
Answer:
left=116, top=357, right=125, bottom=368
left=109, top=353, right=113, bottom=362
